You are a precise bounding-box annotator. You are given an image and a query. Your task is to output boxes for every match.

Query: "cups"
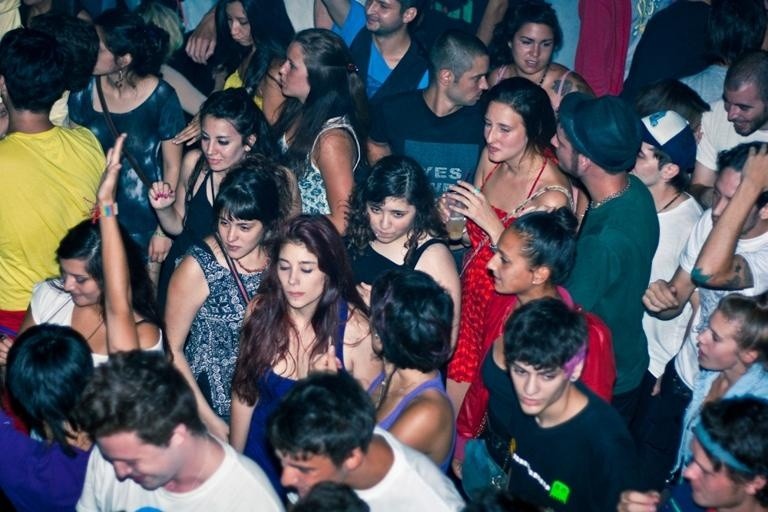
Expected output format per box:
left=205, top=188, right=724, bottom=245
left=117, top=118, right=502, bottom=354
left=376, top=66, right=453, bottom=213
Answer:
left=447, top=193, right=467, bottom=234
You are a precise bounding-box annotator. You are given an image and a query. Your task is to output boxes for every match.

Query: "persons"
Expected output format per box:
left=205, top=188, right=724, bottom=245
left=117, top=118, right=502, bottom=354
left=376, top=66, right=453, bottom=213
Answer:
left=4, top=1, right=768, bottom=510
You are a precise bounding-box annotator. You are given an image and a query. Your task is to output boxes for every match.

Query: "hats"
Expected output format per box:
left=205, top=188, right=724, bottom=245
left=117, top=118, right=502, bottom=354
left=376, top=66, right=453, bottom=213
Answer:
left=557, top=91, right=644, bottom=174
left=641, top=109, right=697, bottom=174
